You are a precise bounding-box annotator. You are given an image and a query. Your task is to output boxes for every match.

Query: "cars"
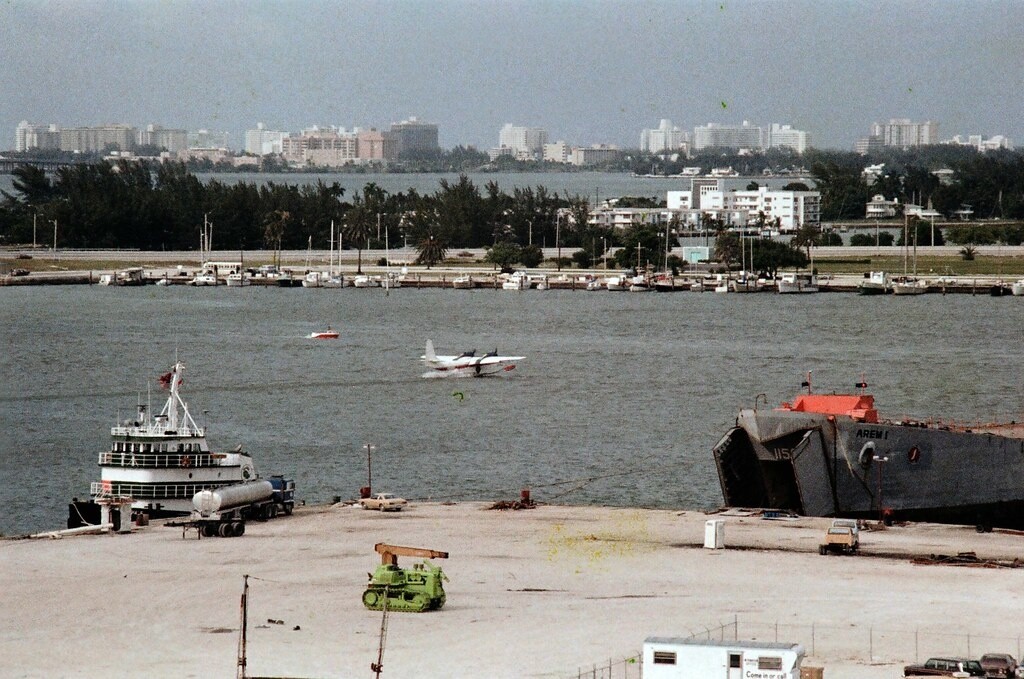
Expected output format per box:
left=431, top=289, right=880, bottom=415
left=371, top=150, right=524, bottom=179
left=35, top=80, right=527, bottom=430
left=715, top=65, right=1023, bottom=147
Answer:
left=904, top=657, right=985, bottom=679
left=359, top=493, right=408, bottom=512
left=1015, top=659, right=1024, bottom=679
left=258, top=265, right=276, bottom=274
left=980, top=653, right=1016, bottom=679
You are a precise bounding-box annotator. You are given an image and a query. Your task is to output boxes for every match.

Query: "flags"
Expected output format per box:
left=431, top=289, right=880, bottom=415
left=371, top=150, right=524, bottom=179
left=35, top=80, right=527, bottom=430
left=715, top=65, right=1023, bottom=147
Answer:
left=159, top=374, right=172, bottom=389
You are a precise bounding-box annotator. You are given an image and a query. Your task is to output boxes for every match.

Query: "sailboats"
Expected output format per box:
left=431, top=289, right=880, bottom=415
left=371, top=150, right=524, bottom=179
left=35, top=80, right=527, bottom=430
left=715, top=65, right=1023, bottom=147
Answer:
left=301, top=220, right=401, bottom=288
left=587, top=208, right=763, bottom=293
left=891, top=209, right=929, bottom=295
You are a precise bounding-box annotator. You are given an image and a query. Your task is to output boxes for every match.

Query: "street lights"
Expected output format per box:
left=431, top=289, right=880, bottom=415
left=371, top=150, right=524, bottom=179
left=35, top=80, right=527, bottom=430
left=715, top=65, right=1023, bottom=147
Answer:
left=363, top=444, right=374, bottom=488
left=34, top=214, right=44, bottom=250
left=49, top=220, right=57, bottom=259
left=526, top=219, right=531, bottom=244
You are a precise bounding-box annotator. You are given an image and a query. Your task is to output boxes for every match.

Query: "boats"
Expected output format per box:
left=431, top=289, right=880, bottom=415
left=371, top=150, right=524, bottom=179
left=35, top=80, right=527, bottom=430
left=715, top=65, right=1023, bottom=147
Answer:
left=502, top=272, right=532, bottom=290
left=312, top=326, right=339, bottom=339
left=779, top=273, right=819, bottom=294
left=99, top=267, right=147, bottom=286
left=711, top=370, right=1024, bottom=532
left=421, top=339, right=528, bottom=377
left=67, top=361, right=258, bottom=529
left=225, top=274, right=251, bottom=286
left=186, top=276, right=223, bottom=286
left=857, top=272, right=895, bottom=296
left=453, top=276, right=476, bottom=289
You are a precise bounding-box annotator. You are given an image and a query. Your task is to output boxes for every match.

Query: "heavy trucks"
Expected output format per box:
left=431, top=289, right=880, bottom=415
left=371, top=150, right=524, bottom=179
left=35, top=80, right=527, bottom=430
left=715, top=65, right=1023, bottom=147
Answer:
left=191, top=475, right=296, bottom=523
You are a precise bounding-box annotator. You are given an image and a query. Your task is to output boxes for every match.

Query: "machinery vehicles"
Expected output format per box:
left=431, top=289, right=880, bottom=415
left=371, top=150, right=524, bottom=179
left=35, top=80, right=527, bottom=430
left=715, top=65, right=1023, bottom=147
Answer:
left=362, top=542, right=450, bottom=612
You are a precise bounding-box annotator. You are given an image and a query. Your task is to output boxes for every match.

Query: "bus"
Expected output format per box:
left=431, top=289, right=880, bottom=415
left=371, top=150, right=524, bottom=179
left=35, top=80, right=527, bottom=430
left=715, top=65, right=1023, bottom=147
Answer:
left=203, top=262, right=242, bottom=276
